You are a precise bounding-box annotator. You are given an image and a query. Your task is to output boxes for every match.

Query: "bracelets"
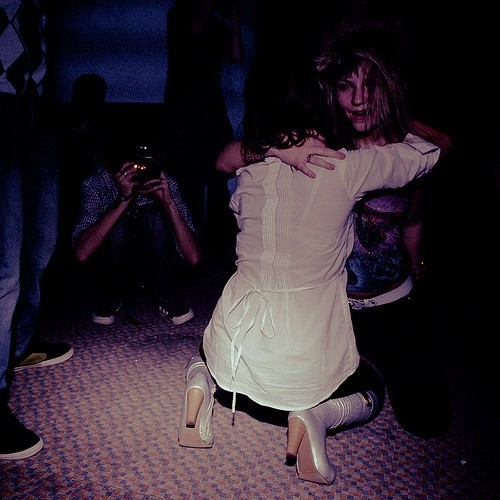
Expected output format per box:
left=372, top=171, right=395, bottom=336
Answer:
left=243, top=144, right=256, bottom=163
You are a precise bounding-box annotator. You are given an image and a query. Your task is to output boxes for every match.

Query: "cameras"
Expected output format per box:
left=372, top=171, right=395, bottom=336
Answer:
left=129, top=146, right=162, bottom=196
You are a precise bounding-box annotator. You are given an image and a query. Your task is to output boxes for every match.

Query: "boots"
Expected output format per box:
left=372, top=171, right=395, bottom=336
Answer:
left=178, top=358, right=216, bottom=447
left=285, top=393, right=366, bottom=484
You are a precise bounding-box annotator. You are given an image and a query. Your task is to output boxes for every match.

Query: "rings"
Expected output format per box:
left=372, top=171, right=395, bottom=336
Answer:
left=308, top=155, right=311, bottom=161
left=119, top=168, right=127, bottom=178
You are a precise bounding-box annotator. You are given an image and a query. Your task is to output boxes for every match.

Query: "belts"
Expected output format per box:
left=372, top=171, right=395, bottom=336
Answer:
left=347, top=277, right=413, bottom=310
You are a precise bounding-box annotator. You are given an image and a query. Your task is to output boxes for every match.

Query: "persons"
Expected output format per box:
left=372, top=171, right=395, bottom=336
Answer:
left=0, top=1, right=75, bottom=460
left=71, top=137, right=201, bottom=325
left=178, top=47, right=450, bottom=484
left=217, top=29, right=430, bottom=434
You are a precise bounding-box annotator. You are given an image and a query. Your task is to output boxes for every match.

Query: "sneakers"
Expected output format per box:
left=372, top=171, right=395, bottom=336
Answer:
left=159, top=307, right=195, bottom=325
left=92, top=304, right=121, bottom=325
left=13, top=337, right=74, bottom=372
left=0, top=406, right=44, bottom=460
